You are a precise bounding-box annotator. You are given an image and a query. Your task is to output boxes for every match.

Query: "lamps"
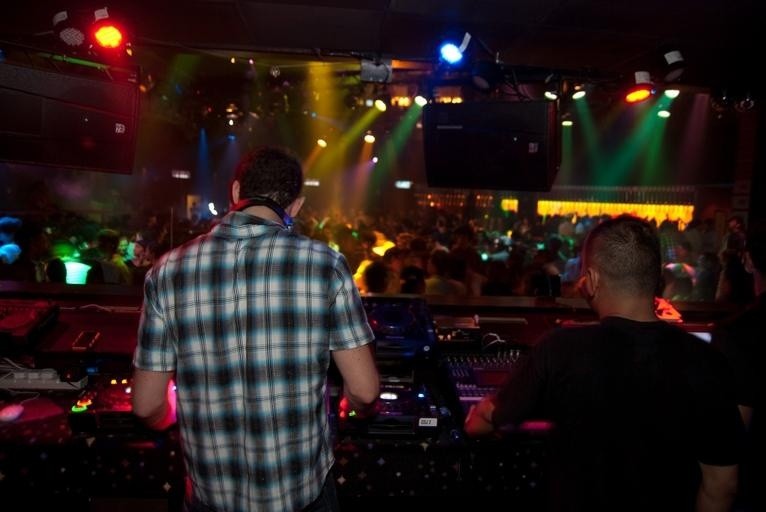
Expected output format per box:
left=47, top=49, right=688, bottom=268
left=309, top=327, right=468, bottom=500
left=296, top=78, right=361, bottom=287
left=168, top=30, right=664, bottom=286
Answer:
left=412, top=79, right=435, bottom=108
left=436, top=27, right=478, bottom=65
left=372, top=86, right=394, bottom=114
left=543, top=68, right=589, bottom=129
left=625, top=47, right=684, bottom=105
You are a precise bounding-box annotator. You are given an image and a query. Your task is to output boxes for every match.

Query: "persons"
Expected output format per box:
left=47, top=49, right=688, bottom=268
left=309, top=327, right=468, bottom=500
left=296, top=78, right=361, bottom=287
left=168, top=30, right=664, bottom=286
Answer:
left=709, top=199, right=766, bottom=511
left=0, top=201, right=214, bottom=286
left=127, top=149, right=381, bottom=512
left=291, top=202, right=611, bottom=305
left=460, top=213, right=745, bottom=511
left=654, top=217, right=744, bottom=304
